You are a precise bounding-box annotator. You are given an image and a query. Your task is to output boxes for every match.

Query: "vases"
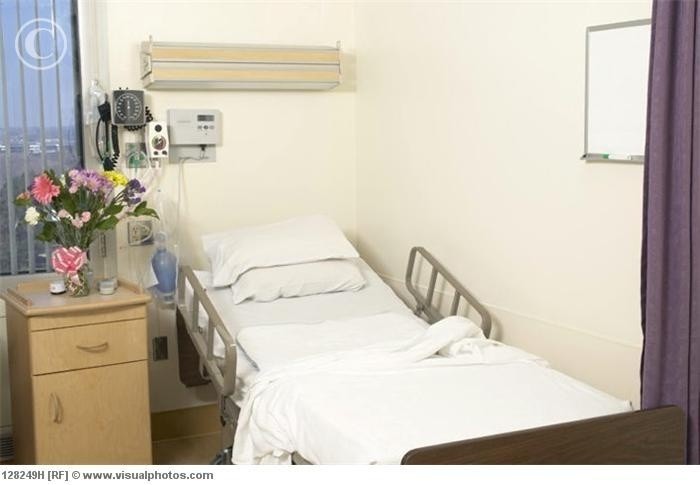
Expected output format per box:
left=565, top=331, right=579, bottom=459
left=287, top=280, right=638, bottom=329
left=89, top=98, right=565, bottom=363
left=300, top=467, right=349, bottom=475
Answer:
left=56, top=264, right=92, bottom=297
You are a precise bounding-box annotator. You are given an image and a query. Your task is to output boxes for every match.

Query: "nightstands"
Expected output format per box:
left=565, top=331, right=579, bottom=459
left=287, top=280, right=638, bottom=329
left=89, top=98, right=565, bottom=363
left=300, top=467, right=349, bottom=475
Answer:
left=2, top=282, right=157, bottom=465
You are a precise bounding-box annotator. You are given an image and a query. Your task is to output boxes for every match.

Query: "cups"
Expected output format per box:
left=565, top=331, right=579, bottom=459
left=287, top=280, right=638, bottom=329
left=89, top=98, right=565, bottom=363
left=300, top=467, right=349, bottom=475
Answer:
left=49, top=282, right=66, bottom=294
left=97, top=277, right=118, bottom=295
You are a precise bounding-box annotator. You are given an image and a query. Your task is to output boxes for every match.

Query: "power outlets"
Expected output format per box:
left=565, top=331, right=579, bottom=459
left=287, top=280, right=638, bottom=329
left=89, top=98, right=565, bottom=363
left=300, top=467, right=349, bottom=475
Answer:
left=127, top=220, right=155, bottom=247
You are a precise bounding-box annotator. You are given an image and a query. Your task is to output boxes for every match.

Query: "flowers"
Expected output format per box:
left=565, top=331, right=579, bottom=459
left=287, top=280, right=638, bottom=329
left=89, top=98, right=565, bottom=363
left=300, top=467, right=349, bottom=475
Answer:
left=14, top=167, right=163, bottom=276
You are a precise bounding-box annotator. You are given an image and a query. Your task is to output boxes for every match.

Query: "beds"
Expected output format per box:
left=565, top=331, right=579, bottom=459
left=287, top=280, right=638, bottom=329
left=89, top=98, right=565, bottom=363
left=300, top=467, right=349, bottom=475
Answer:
left=176, top=243, right=693, bottom=463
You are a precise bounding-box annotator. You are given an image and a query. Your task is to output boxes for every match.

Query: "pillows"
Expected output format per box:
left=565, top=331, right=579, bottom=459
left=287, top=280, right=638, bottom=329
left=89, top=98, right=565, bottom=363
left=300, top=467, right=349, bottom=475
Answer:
left=228, top=258, right=369, bottom=308
left=199, top=215, right=363, bottom=291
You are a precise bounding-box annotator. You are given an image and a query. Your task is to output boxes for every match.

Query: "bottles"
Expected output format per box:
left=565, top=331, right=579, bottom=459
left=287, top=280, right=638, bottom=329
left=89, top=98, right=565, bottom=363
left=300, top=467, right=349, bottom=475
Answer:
left=151, top=246, right=178, bottom=293
